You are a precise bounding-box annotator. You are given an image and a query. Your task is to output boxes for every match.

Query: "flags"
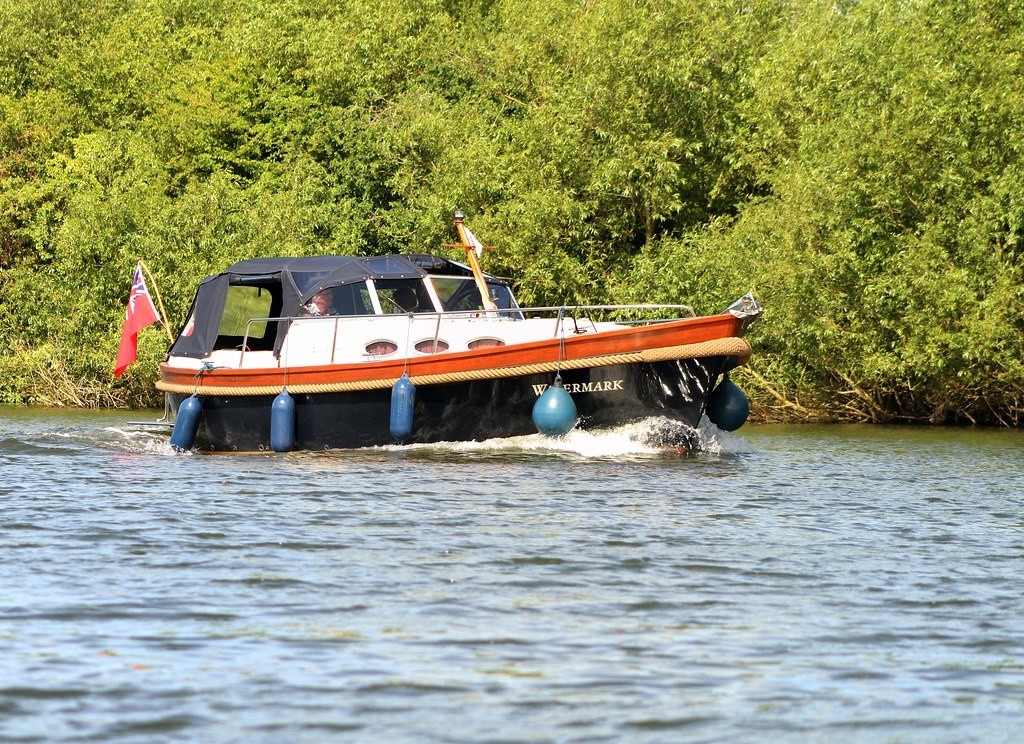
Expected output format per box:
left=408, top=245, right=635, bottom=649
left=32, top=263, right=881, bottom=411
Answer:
left=114, top=266, right=160, bottom=378
left=464, top=226, right=483, bottom=258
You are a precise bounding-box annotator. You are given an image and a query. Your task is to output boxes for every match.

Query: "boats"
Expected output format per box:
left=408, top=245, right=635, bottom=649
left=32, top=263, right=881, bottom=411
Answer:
left=115, top=211, right=763, bottom=452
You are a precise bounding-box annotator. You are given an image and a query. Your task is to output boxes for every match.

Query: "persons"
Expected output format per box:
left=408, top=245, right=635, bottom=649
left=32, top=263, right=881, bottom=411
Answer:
left=392, top=288, right=418, bottom=313
left=301, top=288, right=340, bottom=316
